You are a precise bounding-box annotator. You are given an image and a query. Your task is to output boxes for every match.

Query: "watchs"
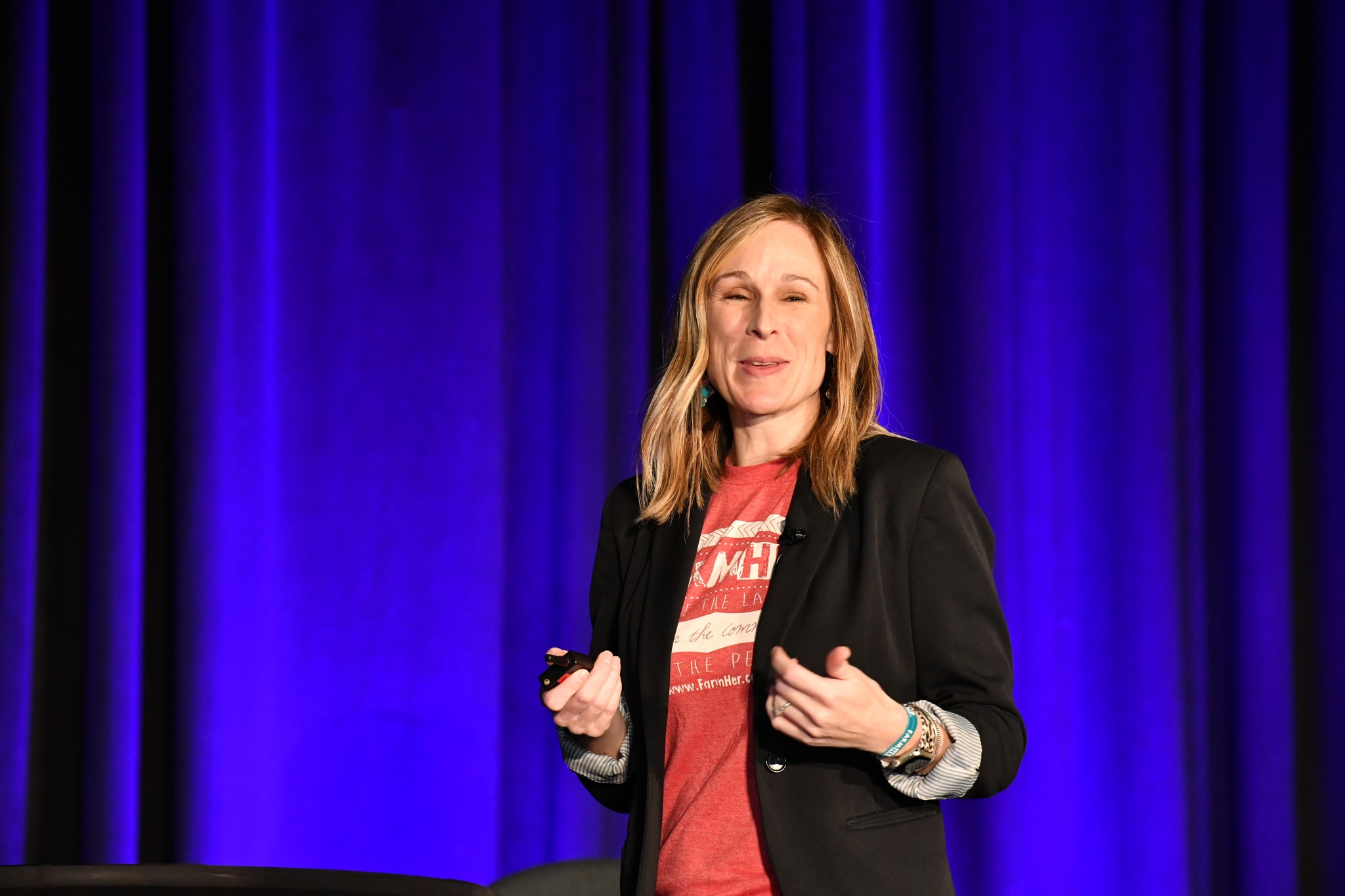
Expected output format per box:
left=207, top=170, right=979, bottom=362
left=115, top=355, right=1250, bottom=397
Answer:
left=889, top=704, right=936, bottom=777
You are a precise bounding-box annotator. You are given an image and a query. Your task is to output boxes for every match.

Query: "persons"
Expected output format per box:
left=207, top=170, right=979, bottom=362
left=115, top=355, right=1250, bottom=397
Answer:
left=543, top=196, right=1036, bottom=896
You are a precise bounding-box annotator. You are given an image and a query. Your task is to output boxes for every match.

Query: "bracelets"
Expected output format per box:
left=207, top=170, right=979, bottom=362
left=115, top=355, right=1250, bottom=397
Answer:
left=881, top=704, right=920, bottom=760
left=911, top=707, right=942, bottom=773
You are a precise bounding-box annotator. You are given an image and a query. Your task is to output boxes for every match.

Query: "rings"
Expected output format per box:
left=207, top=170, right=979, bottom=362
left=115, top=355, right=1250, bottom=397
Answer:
left=774, top=701, right=793, bottom=716
